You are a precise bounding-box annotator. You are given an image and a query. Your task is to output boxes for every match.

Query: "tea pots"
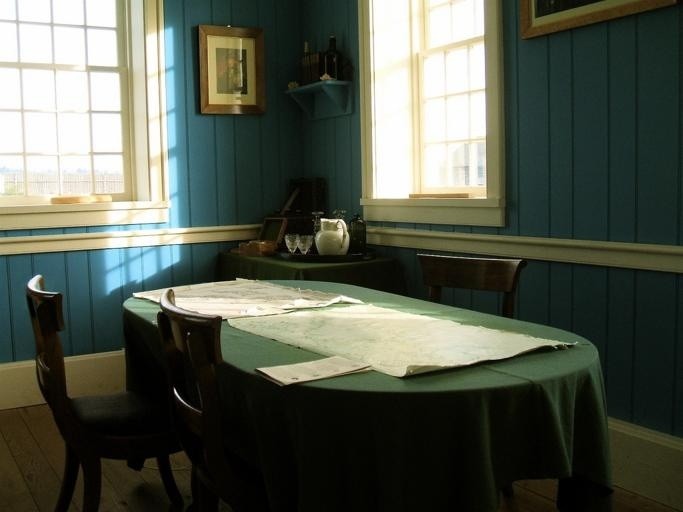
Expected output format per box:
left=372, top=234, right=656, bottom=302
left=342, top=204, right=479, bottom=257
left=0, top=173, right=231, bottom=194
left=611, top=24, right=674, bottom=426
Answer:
left=315, top=217, right=350, bottom=255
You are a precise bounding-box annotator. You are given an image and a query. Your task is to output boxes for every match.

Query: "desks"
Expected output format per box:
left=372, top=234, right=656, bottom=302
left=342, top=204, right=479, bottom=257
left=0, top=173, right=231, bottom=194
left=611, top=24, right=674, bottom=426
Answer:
left=121, top=278, right=613, bottom=509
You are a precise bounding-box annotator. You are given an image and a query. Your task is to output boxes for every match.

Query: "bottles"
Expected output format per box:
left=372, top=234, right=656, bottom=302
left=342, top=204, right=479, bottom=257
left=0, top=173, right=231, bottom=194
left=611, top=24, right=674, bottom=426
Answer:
left=350, top=214, right=366, bottom=253
left=301, top=33, right=342, bottom=84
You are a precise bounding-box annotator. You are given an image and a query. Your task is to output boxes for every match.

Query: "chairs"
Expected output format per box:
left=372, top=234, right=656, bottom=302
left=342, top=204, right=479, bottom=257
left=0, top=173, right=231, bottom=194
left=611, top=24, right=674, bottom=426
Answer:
left=156, top=299, right=252, bottom=509
left=25, top=271, right=187, bottom=510
left=414, top=256, right=525, bottom=319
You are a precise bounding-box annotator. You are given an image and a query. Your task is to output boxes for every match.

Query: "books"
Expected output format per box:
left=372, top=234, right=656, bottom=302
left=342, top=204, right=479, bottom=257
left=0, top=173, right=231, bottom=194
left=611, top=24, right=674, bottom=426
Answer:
left=253, top=352, right=372, bottom=389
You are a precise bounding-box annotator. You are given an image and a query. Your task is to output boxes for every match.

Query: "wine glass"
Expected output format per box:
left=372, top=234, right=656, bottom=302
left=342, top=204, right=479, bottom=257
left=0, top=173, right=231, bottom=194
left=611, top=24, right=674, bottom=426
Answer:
left=285, top=233, right=312, bottom=256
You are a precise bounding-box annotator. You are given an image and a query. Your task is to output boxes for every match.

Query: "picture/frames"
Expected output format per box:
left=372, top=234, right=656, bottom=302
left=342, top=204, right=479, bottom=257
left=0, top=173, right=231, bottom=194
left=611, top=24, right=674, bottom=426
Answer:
left=192, top=23, right=270, bottom=116
left=516, top=1, right=681, bottom=41
